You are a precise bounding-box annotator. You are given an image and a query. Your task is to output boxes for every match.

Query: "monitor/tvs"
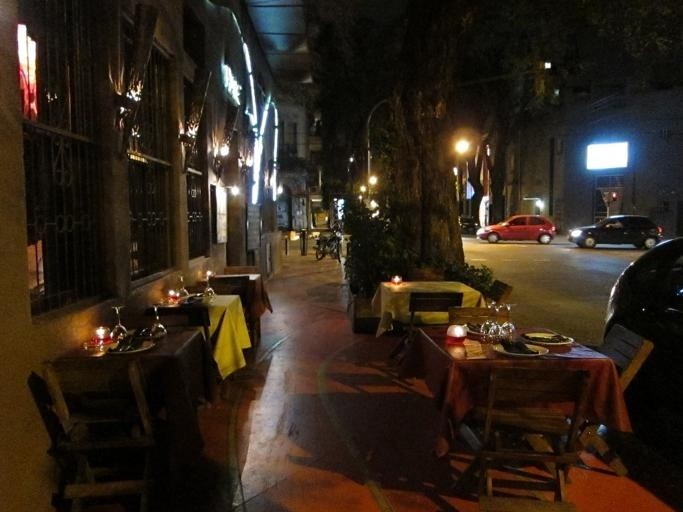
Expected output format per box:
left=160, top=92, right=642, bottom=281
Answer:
left=586, top=140, right=629, bottom=172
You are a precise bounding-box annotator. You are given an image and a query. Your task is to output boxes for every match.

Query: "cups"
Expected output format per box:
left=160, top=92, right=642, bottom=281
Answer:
left=446, top=324, right=467, bottom=339
left=447, top=345, right=465, bottom=360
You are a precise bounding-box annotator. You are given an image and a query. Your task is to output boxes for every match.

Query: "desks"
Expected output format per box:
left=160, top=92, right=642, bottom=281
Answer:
left=185, top=274, right=272, bottom=344
left=156, top=293, right=252, bottom=380
left=61, top=325, right=225, bottom=418
left=371, top=280, right=494, bottom=366
left=414, top=324, right=633, bottom=471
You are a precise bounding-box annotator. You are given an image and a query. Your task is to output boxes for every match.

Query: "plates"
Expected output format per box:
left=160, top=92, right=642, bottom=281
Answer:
left=492, top=342, right=549, bottom=357
left=104, top=338, right=156, bottom=355
left=518, top=331, right=574, bottom=348
left=177, top=293, right=203, bottom=308
left=461, top=322, right=509, bottom=337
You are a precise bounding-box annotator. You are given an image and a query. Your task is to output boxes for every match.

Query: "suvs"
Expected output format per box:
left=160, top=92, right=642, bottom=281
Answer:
left=567, top=214, right=663, bottom=251
left=457, top=214, right=478, bottom=235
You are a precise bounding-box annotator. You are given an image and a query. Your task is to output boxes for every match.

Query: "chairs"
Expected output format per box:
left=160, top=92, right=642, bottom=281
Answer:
left=541, top=322, right=658, bottom=479
left=452, top=366, right=593, bottom=501
left=482, top=281, right=514, bottom=307
left=40, top=359, right=162, bottom=511
left=389, top=290, right=464, bottom=361
left=208, top=276, right=258, bottom=347
left=146, top=304, right=223, bottom=408
left=223, top=266, right=259, bottom=274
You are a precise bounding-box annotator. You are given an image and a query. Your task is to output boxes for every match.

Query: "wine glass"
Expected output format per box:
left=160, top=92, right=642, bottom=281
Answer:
left=480, top=299, right=496, bottom=334
left=199, top=270, right=216, bottom=300
left=148, top=302, right=168, bottom=339
left=175, top=272, right=189, bottom=297
left=498, top=303, right=518, bottom=337
left=489, top=302, right=506, bottom=340
left=107, top=304, right=130, bottom=340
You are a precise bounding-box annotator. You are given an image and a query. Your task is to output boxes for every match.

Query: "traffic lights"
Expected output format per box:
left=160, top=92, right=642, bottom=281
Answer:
left=612, top=191, right=617, bottom=201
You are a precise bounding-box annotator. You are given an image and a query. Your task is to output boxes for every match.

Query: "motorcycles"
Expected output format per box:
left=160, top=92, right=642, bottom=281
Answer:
left=312, top=224, right=341, bottom=264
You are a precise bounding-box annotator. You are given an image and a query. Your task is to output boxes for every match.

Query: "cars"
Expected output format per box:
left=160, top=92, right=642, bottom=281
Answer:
left=476, top=214, right=555, bottom=243
left=601, top=236, right=682, bottom=468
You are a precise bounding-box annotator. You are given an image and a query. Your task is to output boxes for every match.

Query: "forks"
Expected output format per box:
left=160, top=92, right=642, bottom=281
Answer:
left=120, top=326, right=149, bottom=351
